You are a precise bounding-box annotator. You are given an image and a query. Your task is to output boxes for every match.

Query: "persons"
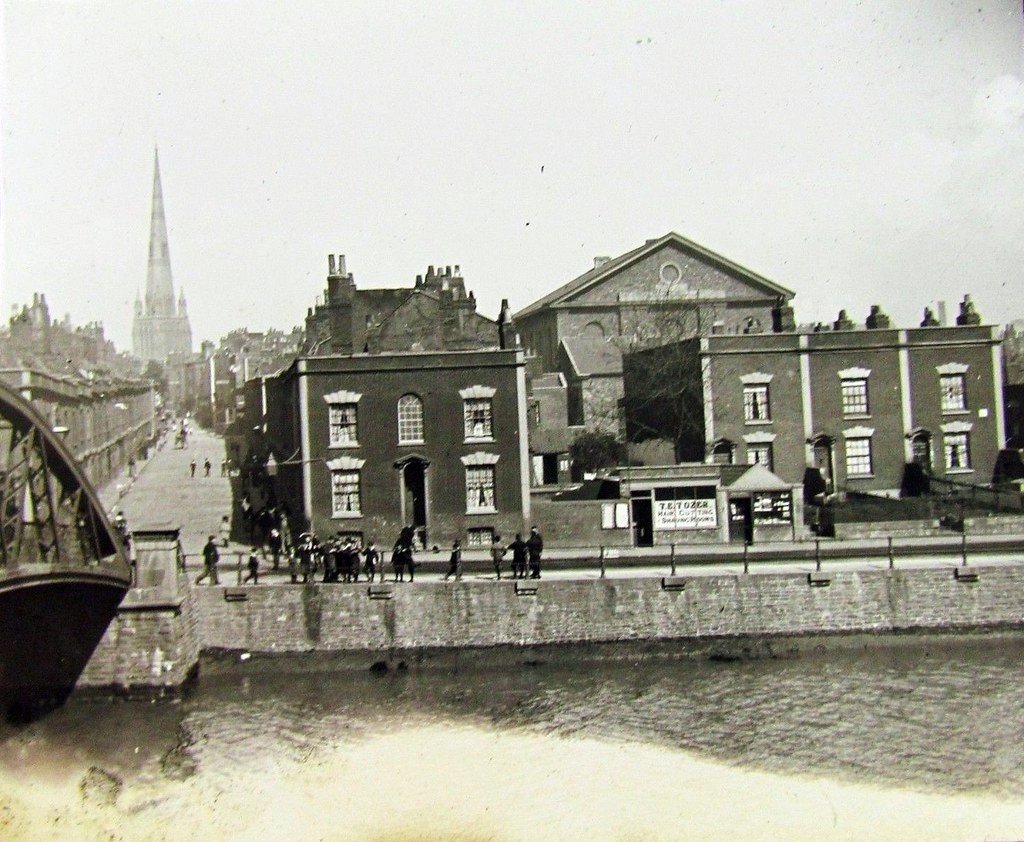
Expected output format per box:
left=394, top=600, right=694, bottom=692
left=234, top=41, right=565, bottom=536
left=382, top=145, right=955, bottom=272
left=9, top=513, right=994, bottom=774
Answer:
left=270, top=528, right=282, bottom=569
left=490, top=535, right=507, bottom=580
left=241, top=452, right=295, bottom=556
left=191, top=460, right=196, bottom=477
left=444, top=537, right=464, bottom=582
left=244, top=548, right=259, bottom=584
left=219, top=516, right=231, bottom=547
left=506, top=533, right=530, bottom=580
left=204, top=458, right=211, bottom=478
left=111, top=512, right=127, bottom=534
left=288, top=532, right=360, bottom=583
left=523, top=525, right=544, bottom=579
left=390, top=544, right=415, bottom=583
left=221, top=458, right=232, bottom=476
left=361, top=541, right=379, bottom=584
left=193, top=535, right=220, bottom=586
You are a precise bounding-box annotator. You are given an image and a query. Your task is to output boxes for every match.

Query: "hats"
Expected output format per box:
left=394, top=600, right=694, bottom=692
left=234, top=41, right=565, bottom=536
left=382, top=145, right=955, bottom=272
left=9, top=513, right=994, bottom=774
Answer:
left=208, top=536, right=215, bottom=540
